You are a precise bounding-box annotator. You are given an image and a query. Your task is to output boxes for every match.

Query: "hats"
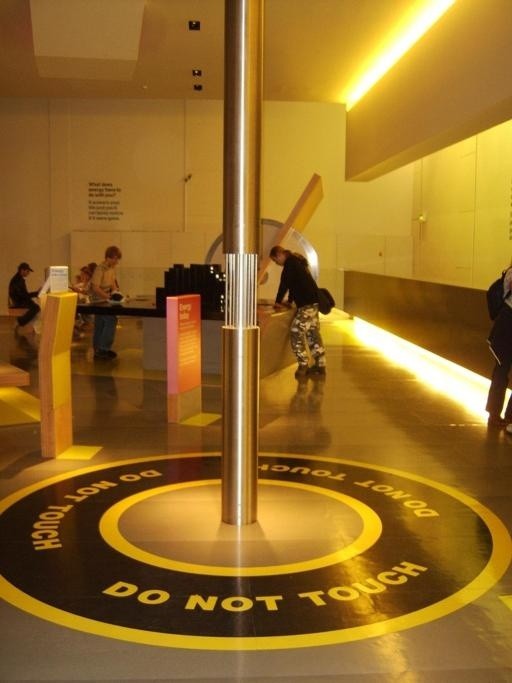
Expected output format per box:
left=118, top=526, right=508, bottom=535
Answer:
left=18, top=263, right=32, bottom=272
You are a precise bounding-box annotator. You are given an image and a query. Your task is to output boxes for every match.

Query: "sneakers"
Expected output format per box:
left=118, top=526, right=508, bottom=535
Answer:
left=296, top=365, right=325, bottom=376
left=94, top=349, right=116, bottom=360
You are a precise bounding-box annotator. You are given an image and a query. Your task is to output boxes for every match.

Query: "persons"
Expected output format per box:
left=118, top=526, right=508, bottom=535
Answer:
left=279, top=377, right=331, bottom=454
left=69, top=245, right=122, bottom=360
left=10, top=262, right=42, bottom=328
left=477, top=426, right=511, bottom=560
left=85, top=362, right=118, bottom=418
left=7, top=328, right=38, bottom=371
left=269, top=245, right=327, bottom=377
left=486, top=265, right=511, bottom=426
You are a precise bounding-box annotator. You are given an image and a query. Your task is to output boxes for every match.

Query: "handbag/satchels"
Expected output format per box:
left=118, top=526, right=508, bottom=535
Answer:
left=316, top=287, right=335, bottom=314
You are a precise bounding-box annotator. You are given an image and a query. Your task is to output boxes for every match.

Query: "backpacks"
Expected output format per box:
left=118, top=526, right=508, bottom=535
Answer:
left=486, top=267, right=512, bottom=320
